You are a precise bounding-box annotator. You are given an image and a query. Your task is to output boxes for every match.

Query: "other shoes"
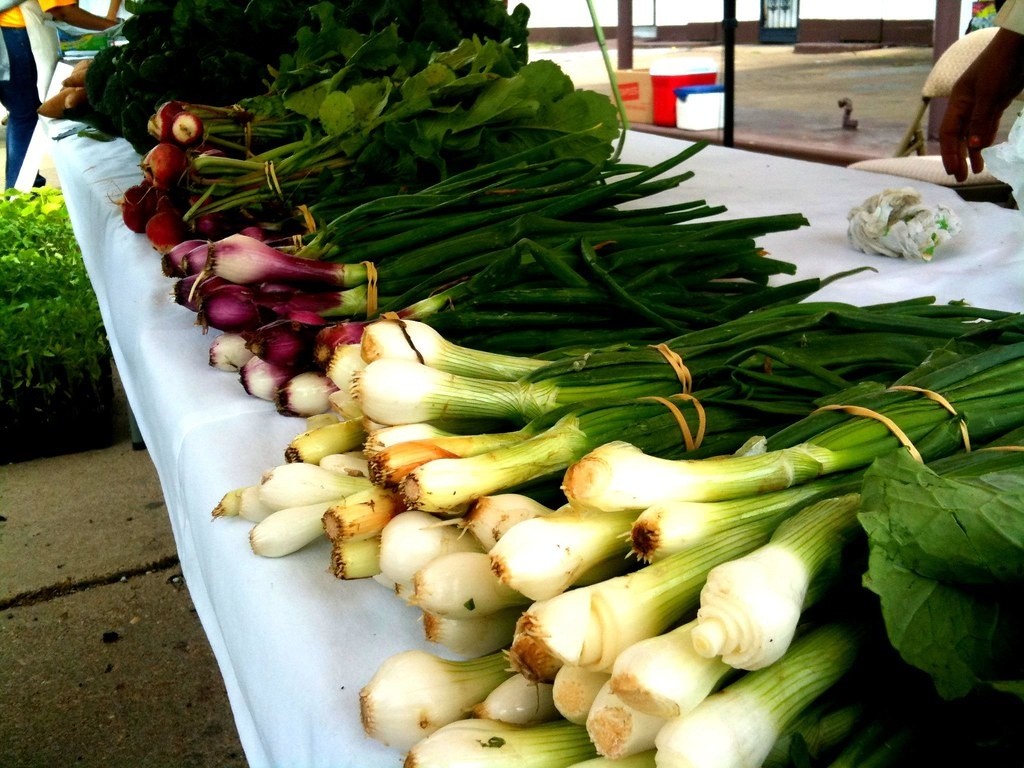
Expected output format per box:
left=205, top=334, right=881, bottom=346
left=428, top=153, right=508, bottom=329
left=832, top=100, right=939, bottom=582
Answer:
left=33, top=174, right=46, bottom=187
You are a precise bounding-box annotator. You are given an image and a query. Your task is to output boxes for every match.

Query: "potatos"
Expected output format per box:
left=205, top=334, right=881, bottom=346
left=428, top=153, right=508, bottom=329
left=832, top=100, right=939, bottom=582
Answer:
left=36, top=60, right=91, bottom=118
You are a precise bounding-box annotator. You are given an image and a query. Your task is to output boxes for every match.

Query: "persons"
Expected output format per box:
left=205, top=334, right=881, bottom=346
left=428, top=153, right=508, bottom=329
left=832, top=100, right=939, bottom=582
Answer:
left=0, top=0, right=138, bottom=195
left=941, top=0, right=1024, bottom=182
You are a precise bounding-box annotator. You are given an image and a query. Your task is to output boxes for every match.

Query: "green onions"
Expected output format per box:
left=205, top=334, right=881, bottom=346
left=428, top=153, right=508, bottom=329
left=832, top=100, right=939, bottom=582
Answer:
left=161, top=125, right=1024, bottom=768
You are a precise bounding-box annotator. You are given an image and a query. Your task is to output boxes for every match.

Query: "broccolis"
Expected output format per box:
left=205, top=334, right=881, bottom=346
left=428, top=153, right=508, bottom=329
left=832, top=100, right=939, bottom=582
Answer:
left=60, top=0, right=532, bottom=156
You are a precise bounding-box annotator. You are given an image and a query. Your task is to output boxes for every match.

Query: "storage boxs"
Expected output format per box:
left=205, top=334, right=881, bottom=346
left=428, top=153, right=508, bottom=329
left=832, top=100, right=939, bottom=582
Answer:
left=672, top=85, right=724, bottom=131
left=648, top=56, right=720, bottom=127
left=611, top=68, right=653, bottom=124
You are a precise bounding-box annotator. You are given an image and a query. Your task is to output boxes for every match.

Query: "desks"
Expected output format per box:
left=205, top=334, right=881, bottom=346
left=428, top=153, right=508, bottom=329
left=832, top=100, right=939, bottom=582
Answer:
left=43, top=62, right=1023, bottom=768
